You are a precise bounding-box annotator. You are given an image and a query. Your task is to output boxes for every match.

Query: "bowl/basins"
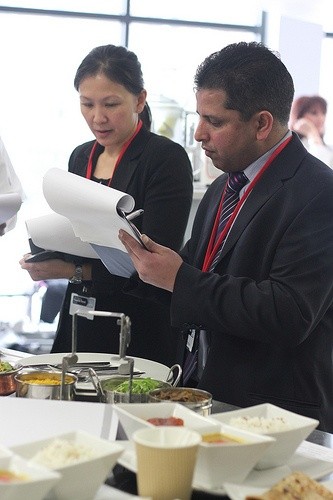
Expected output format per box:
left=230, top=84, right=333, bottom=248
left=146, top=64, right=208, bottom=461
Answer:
left=194, top=423, right=276, bottom=490
left=0, top=360, right=23, bottom=395
left=148, top=387, right=213, bottom=415
left=14, top=371, right=77, bottom=401
left=116, top=401, right=215, bottom=440
left=213, top=403, right=319, bottom=471
left=88, top=365, right=182, bottom=404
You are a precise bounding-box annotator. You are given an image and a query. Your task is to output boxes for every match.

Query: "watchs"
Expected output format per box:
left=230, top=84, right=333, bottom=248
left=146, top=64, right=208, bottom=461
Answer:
left=70, top=263, right=83, bottom=284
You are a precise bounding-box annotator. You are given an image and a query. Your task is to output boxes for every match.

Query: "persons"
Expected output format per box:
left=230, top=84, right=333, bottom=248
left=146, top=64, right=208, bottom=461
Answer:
left=120, top=42, right=333, bottom=438
left=17, top=43, right=194, bottom=387
left=290, top=96, right=333, bottom=169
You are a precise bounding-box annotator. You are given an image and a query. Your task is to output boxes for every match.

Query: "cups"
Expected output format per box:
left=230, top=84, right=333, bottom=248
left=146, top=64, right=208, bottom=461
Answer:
left=0, top=430, right=125, bottom=499
left=134, top=428, right=201, bottom=500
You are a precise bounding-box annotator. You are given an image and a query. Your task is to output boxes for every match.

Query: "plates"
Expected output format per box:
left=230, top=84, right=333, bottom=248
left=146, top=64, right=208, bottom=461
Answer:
left=95, top=482, right=140, bottom=499
left=17, top=352, right=174, bottom=391
left=117, top=438, right=333, bottom=493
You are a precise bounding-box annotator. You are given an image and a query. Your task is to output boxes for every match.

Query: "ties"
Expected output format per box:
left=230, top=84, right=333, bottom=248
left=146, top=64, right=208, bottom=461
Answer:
left=173, top=170, right=251, bottom=389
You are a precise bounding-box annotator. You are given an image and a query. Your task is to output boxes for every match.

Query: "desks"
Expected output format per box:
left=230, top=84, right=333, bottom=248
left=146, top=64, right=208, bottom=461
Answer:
left=0, top=350, right=333, bottom=500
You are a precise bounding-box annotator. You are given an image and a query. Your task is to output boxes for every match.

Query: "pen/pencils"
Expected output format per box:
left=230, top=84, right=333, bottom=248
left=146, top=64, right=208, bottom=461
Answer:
left=127, top=209, right=144, bottom=221
